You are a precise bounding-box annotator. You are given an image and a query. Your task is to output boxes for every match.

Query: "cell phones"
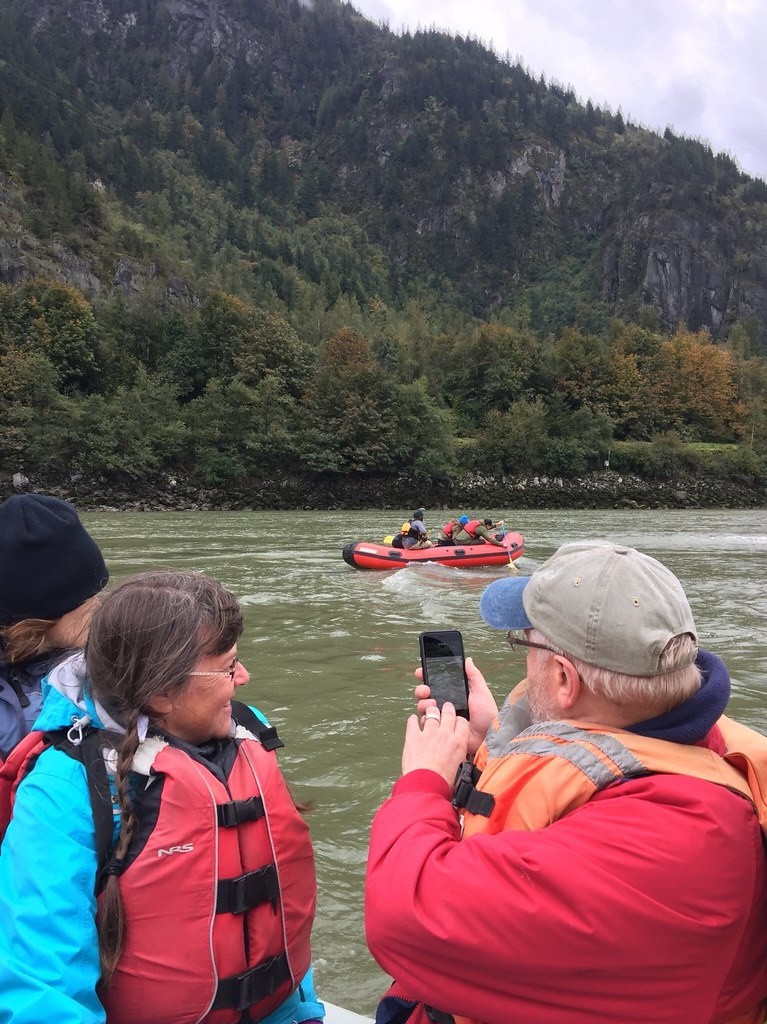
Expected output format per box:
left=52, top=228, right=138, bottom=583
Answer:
left=418, top=630, right=470, bottom=720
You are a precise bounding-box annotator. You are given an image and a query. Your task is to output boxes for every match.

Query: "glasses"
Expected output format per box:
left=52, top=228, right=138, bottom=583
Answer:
left=184, top=657, right=239, bottom=682
left=504, top=630, right=583, bottom=682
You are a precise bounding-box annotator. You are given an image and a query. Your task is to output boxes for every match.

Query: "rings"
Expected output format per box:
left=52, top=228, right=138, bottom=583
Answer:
left=424, top=713, right=441, bottom=723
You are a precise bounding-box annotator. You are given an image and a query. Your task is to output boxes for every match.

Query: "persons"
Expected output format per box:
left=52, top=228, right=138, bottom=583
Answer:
left=365, top=541, right=766, bottom=1023
left=389, top=510, right=509, bottom=548
left=0, top=570, right=325, bottom=1024
left=0, top=495, right=109, bottom=760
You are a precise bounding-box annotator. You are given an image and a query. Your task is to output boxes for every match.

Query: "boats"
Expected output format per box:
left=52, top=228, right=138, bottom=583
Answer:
left=342, top=530, right=524, bottom=571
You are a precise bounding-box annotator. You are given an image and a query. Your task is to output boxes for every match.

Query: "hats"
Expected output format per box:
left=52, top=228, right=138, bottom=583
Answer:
left=413, top=510, right=426, bottom=518
left=460, top=515, right=469, bottom=524
left=0, top=494, right=110, bottom=619
left=479, top=539, right=698, bottom=677
left=484, top=518, right=493, bottom=527
